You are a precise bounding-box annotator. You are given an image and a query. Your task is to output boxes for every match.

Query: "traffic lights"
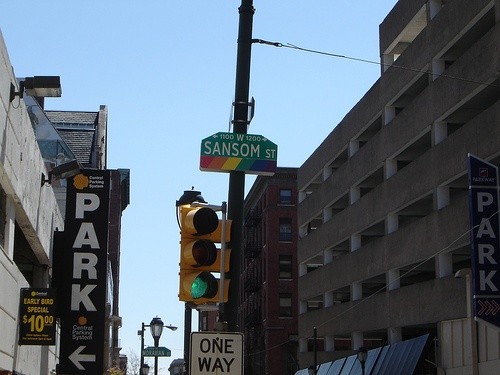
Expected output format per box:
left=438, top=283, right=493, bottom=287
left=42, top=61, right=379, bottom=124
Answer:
left=175, top=186, right=233, bottom=304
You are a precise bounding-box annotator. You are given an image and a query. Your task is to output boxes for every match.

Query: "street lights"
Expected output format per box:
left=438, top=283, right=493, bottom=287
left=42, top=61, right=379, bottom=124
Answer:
left=141, top=318, right=178, bottom=375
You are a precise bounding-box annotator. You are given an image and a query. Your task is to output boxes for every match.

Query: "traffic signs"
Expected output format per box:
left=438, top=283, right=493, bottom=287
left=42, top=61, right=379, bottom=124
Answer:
left=17, top=288, right=58, bottom=346
left=58, top=168, right=110, bottom=375
left=188, top=332, right=245, bottom=375
left=198, top=131, right=278, bottom=176
left=142, top=347, right=171, bottom=358
left=467, top=153, right=500, bottom=332
